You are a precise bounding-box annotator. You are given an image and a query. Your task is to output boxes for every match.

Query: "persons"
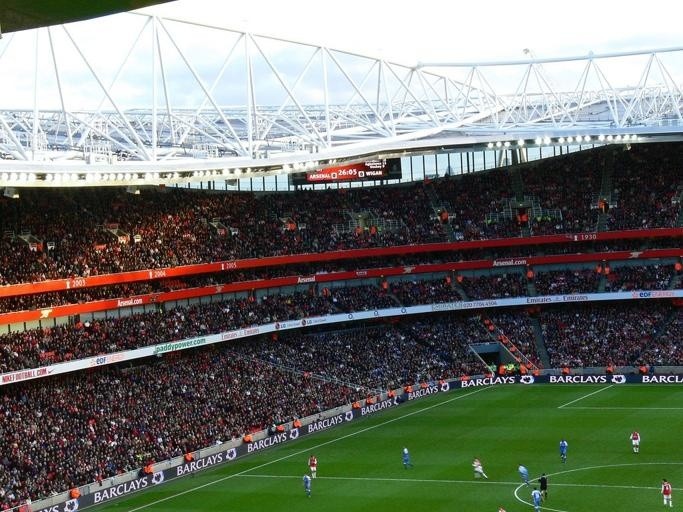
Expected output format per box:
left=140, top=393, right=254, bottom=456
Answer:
left=1, top=184, right=253, bottom=508
left=517, top=463, right=530, bottom=486
left=401, top=445, right=411, bottom=469
left=661, top=478, right=673, bottom=508
left=308, top=455, right=317, bottom=479
left=560, top=439, right=568, bottom=462
left=539, top=473, right=548, bottom=498
left=303, top=474, right=311, bottom=498
left=630, top=430, right=641, bottom=455
left=473, top=458, right=488, bottom=479
left=532, top=488, right=541, bottom=510
left=463, top=141, right=683, bottom=378
left=249, top=184, right=479, bottom=427
